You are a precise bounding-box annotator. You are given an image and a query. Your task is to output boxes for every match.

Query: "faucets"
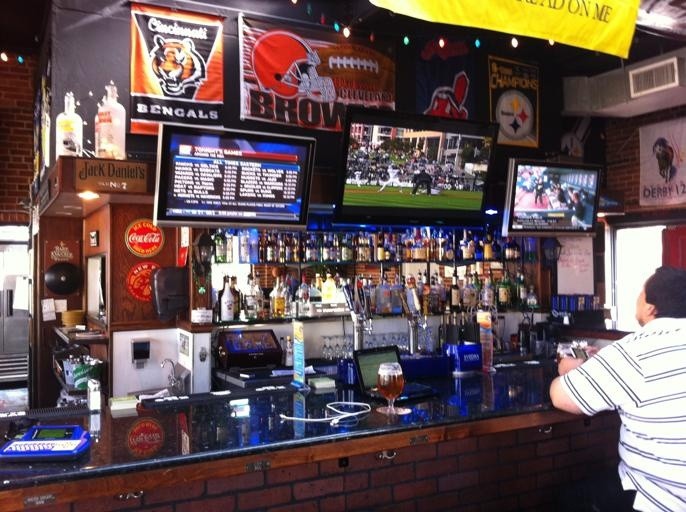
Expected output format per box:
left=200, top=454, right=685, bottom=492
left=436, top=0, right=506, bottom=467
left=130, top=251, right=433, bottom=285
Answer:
left=160, top=358, right=175, bottom=387
left=401, top=280, right=428, bottom=355
left=343, top=280, right=372, bottom=349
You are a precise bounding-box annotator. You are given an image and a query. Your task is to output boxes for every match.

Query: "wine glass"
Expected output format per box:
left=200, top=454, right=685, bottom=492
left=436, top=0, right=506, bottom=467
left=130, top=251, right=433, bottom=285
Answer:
left=320, top=323, right=436, bottom=361
left=376, top=362, right=405, bottom=415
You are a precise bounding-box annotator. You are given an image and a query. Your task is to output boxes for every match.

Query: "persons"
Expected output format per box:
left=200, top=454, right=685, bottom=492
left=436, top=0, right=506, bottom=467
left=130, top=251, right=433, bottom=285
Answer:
left=534, top=178, right=586, bottom=221
left=548, top=265, right=686, bottom=511
left=346, top=141, right=485, bottom=196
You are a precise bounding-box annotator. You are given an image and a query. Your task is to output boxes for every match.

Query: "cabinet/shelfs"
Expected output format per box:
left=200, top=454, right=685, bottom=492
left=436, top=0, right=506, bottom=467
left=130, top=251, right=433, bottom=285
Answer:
left=53, top=324, right=107, bottom=397
left=176, top=221, right=542, bottom=330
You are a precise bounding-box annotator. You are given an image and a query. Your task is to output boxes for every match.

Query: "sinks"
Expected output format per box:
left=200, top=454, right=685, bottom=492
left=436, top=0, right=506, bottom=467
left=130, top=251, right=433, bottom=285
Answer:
left=127, top=388, right=181, bottom=401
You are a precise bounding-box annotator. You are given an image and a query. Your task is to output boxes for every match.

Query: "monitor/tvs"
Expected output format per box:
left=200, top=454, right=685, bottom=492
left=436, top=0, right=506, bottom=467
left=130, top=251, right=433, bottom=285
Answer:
left=153, top=122, right=318, bottom=234
left=331, top=104, right=502, bottom=227
left=501, top=157, right=605, bottom=240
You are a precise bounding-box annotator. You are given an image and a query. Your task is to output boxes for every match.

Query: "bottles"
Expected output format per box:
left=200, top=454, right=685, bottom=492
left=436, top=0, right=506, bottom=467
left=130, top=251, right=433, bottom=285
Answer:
left=55, top=90, right=84, bottom=162
left=279, top=335, right=293, bottom=367
left=94, top=84, right=126, bottom=162
left=194, top=222, right=524, bottom=266
left=210, top=262, right=539, bottom=322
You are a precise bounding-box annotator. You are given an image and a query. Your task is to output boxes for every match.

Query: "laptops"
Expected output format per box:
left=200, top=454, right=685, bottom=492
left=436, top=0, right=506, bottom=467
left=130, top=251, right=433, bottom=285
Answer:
left=353, top=345, right=437, bottom=404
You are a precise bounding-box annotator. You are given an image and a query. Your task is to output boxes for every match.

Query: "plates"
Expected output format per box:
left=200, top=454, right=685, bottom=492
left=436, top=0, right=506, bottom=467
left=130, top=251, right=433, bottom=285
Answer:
left=61, top=309, right=87, bottom=328
